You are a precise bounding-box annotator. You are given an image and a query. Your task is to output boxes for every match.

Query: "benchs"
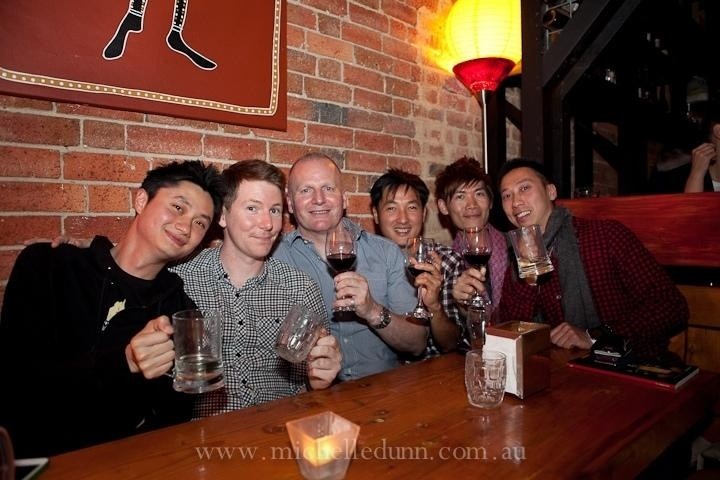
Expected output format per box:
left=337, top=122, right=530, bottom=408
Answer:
left=508, top=192, right=720, bottom=479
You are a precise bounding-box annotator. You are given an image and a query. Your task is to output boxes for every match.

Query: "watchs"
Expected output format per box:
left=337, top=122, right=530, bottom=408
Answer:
left=373, top=302, right=392, bottom=329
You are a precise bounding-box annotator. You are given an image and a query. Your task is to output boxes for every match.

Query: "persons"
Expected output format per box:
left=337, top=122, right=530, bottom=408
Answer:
left=684, top=121, right=720, bottom=193
left=0, top=158, right=227, bottom=464
left=434, top=155, right=511, bottom=325
left=23, top=157, right=343, bottom=429
left=488, top=156, right=689, bottom=354
left=271, top=150, right=431, bottom=390
left=369, top=166, right=467, bottom=370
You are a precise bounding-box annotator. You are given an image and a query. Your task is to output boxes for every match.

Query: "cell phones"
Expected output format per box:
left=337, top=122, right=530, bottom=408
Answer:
left=14, top=456, right=49, bottom=480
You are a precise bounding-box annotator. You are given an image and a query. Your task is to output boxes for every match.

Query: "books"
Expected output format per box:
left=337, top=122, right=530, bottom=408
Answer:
left=563, top=346, right=701, bottom=390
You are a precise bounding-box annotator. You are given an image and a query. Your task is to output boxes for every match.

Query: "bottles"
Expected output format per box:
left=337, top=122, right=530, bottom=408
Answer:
left=542, top=8, right=571, bottom=31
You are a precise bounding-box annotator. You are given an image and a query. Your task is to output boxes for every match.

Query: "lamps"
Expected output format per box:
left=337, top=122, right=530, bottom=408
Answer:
left=445, top=0, right=521, bottom=176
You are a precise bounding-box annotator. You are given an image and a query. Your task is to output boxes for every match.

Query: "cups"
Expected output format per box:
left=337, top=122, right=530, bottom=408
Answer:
left=465, top=348, right=507, bottom=410
left=163, top=308, right=225, bottom=396
left=274, top=303, right=326, bottom=365
left=507, top=223, right=556, bottom=280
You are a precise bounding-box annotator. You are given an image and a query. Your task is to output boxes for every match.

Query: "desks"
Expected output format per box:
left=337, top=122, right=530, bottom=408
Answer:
left=33, top=350, right=711, bottom=480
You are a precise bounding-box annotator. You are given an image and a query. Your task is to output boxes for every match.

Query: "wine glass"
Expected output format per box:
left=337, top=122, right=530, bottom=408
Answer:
left=325, top=228, right=357, bottom=313
left=404, top=237, right=436, bottom=319
left=463, top=226, right=493, bottom=307
